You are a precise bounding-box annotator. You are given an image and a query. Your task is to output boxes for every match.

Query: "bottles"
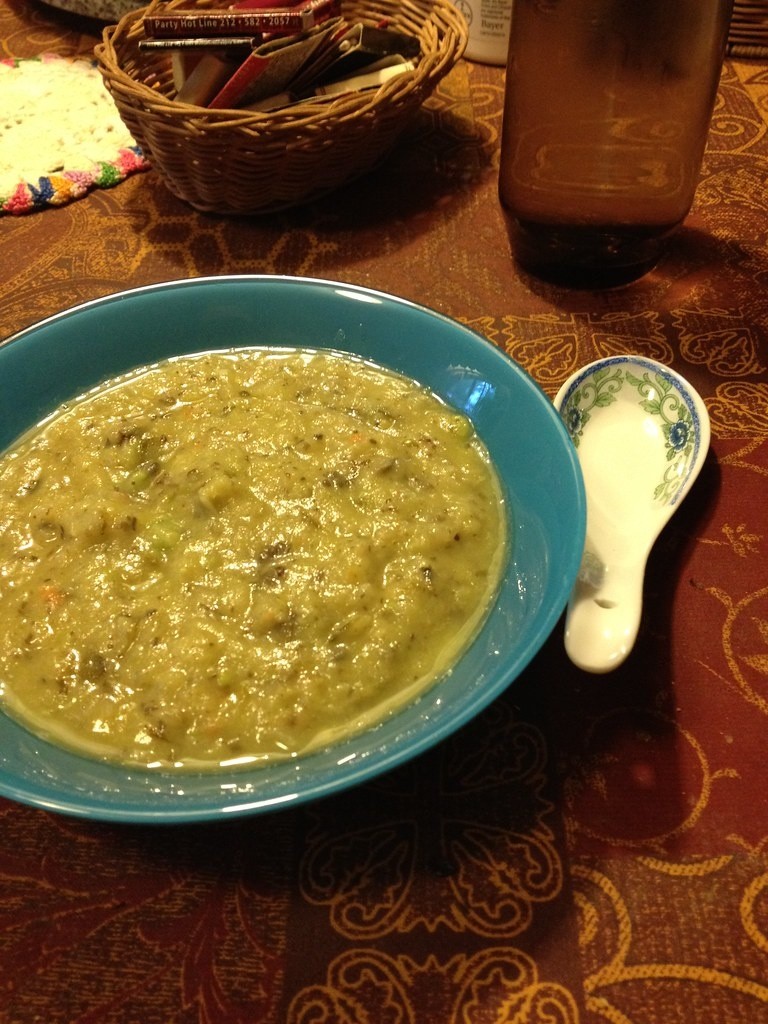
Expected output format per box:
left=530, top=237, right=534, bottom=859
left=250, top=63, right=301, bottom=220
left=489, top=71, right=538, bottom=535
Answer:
left=497, top=0, right=735, bottom=293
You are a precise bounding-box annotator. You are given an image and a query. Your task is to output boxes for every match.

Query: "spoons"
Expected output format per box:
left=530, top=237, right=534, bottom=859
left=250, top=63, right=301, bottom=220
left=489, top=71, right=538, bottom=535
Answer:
left=552, top=355, right=710, bottom=673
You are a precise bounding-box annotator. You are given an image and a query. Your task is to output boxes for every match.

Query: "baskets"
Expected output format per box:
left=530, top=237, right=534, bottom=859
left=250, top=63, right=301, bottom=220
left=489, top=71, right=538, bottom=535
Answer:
left=92, top=0, right=471, bottom=216
left=726, top=0, right=768, bottom=58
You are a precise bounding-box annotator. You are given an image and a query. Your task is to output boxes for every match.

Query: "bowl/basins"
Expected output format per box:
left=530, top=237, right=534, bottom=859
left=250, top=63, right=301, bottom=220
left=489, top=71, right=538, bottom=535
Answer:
left=0, top=273, right=587, bottom=824
